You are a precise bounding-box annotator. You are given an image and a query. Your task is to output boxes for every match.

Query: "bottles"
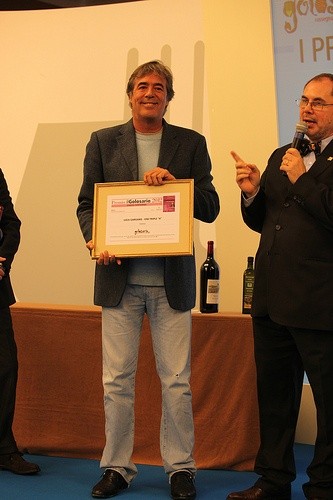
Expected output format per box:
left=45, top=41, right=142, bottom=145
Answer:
left=200, top=241, right=220, bottom=312
left=243, top=256, right=256, bottom=315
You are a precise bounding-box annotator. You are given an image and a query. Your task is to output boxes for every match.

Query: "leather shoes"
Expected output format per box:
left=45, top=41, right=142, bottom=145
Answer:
left=227, top=485, right=272, bottom=500
left=0, top=453, right=39, bottom=475
left=170, top=471, right=196, bottom=500
left=91, top=469, right=128, bottom=497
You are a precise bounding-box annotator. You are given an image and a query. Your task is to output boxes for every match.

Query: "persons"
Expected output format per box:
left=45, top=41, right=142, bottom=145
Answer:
left=76, top=60, right=220, bottom=500
left=0, top=167, right=41, bottom=475
left=227, top=73, right=333, bottom=500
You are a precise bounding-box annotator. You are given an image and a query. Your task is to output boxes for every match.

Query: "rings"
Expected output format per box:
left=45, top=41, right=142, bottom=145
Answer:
left=285, top=161, right=288, bottom=165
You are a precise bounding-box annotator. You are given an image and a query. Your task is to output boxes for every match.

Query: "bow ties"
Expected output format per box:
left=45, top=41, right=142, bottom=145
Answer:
left=303, top=143, right=322, bottom=156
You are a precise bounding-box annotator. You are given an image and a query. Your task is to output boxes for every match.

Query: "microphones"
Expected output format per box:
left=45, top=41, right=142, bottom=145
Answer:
left=283, top=121, right=308, bottom=177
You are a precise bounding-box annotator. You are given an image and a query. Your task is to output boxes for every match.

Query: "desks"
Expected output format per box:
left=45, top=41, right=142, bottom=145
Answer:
left=8, top=302, right=262, bottom=471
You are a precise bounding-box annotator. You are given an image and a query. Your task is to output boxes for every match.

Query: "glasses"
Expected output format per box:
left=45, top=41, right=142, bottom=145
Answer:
left=296, top=99, right=333, bottom=112
